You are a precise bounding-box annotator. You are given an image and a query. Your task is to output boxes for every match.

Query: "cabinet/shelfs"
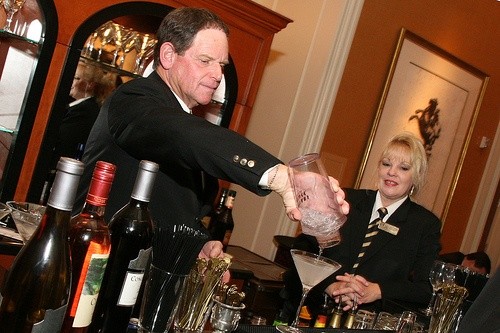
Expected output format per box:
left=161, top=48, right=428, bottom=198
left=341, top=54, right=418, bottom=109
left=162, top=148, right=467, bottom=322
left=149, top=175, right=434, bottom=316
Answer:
left=0, top=0, right=295, bottom=218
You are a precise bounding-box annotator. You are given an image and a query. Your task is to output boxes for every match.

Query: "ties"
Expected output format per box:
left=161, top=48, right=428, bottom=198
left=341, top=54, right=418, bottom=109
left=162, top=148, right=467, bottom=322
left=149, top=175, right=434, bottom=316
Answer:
left=349, top=208, right=388, bottom=274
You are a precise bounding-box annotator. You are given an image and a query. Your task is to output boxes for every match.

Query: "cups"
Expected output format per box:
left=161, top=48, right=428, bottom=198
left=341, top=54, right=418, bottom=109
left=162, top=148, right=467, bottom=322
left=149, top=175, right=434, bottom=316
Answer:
left=0, top=202, right=11, bottom=220
left=164, top=268, right=223, bottom=333
left=137, top=263, right=192, bottom=333
left=210, top=296, right=246, bottom=333
left=5, top=199, right=46, bottom=246
left=428, top=283, right=469, bottom=333
left=449, top=265, right=489, bottom=333
left=350, top=309, right=417, bottom=333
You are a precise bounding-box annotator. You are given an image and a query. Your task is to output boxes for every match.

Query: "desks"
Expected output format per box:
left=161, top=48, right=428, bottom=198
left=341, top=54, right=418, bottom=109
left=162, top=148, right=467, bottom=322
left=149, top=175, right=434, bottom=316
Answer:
left=0, top=218, right=287, bottom=286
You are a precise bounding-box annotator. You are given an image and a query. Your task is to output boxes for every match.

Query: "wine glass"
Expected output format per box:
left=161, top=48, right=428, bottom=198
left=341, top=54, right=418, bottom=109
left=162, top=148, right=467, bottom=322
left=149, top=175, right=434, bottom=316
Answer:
left=82, top=21, right=155, bottom=78
left=275, top=248, right=341, bottom=333
left=3, top=0, right=26, bottom=34
left=417, top=259, right=455, bottom=317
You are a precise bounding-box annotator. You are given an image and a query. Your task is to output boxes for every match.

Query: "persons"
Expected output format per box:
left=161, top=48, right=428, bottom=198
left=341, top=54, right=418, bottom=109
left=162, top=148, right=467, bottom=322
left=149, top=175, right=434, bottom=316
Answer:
left=61, top=56, right=116, bottom=158
left=286, top=134, right=442, bottom=327
left=441, top=251, right=491, bottom=274
left=72, top=7, right=349, bottom=321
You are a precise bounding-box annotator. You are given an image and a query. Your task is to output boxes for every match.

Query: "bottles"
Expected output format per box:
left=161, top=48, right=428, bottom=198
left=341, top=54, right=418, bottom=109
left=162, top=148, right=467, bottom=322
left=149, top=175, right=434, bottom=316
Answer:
left=313, top=304, right=357, bottom=329
left=201, top=186, right=238, bottom=254
left=286, top=153, right=348, bottom=249
left=0, top=155, right=84, bottom=333
left=89, top=159, right=159, bottom=333
left=57, top=159, right=112, bottom=333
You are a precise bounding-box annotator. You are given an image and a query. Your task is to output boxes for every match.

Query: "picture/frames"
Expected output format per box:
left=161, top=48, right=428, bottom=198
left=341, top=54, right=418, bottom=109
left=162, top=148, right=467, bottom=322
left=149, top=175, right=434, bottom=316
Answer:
left=354, top=25, right=491, bottom=237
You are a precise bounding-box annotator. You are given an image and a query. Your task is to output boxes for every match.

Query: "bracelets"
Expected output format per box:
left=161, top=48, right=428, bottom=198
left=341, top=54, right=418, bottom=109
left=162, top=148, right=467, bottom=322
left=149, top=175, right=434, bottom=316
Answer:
left=267, top=167, right=279, bottom=190
